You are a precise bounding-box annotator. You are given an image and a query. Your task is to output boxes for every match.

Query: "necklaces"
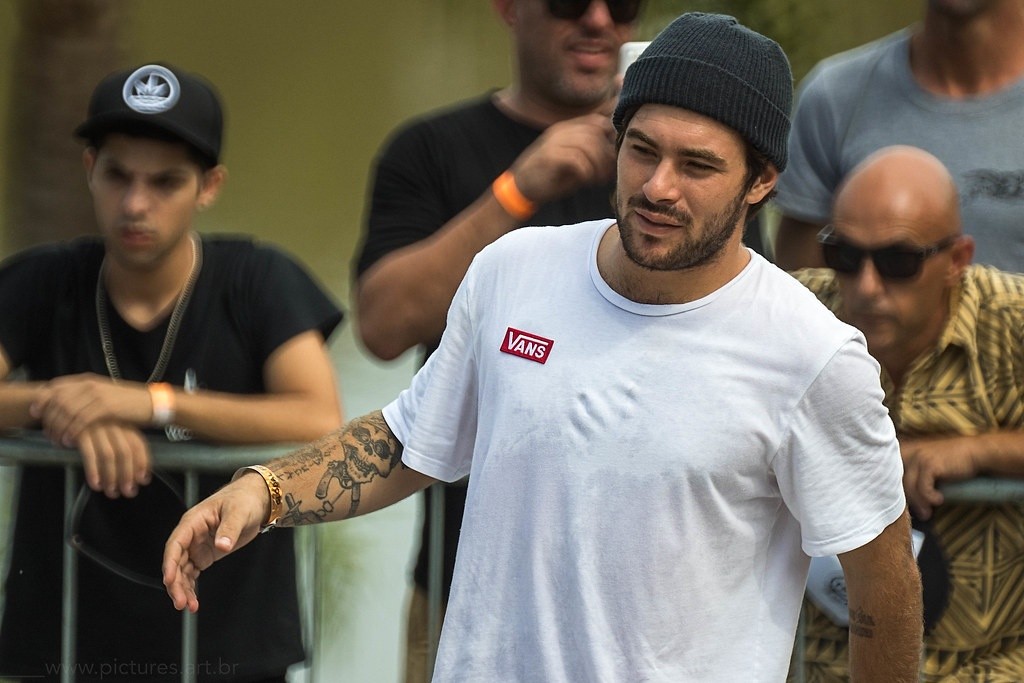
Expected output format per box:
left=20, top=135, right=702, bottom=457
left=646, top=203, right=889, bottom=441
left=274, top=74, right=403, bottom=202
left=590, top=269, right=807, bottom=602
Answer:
left=95, top=231, right=198, bottom=383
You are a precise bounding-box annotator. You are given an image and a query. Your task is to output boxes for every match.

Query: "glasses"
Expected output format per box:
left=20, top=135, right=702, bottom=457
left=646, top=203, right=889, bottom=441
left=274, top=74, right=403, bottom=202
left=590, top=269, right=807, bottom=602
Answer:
left=545, top=0, right=643, bottom=24
left=822, top=235, right=957, bottom=280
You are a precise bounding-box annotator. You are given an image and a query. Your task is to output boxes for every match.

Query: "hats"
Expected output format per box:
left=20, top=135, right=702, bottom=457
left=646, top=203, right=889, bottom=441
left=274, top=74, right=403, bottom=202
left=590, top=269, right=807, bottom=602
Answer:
left=613, top=12, right=793, bottom=173
left=72, top=64, right=221, bottom=167
left=805, top=509, right=952, bottom=640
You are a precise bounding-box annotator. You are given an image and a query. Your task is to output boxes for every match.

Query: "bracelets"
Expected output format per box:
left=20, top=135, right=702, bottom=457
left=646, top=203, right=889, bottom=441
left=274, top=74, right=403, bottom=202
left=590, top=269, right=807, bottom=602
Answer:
left=492, top=170, right=536, bottom=220
left=227, top=464, right=283, bottom=535
left=147, top=381, right=176, bottom=427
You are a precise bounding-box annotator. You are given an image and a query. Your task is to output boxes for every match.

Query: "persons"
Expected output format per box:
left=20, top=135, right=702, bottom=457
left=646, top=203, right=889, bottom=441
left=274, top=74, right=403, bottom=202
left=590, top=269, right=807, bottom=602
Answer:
left=774, top=0, right=1022, bottom=273
left=161, top=13, right=926, bottom=683
left=347, top=0, right=767, bottom=682
left=1, top=60, right=348, bottom=683
left=788, top=146, right=1024, bottom=683
